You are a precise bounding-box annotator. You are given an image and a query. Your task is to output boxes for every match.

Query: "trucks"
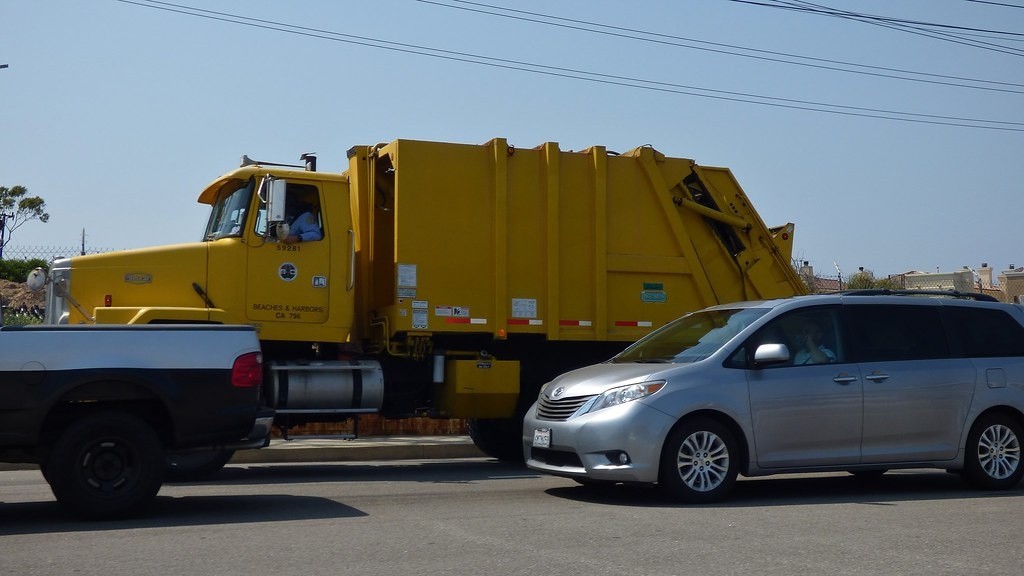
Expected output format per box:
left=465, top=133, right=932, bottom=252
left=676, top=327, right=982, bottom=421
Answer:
left=27, top=137, right=811, bottom=484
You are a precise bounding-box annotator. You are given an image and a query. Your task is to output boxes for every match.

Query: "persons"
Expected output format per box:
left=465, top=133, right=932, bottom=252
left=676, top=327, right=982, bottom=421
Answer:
left=735, top=320, right=836, bottom=365
left=270, top=191, right=322, bottom=243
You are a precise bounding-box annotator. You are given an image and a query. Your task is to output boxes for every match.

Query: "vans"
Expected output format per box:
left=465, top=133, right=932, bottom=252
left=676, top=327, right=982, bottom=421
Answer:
left=521, top=290, right=1024, bottom=506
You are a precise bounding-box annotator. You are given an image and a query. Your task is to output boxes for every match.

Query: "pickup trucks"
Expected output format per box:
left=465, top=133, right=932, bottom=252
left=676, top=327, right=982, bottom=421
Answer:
left=0, top=324, right=272, bottom=522
